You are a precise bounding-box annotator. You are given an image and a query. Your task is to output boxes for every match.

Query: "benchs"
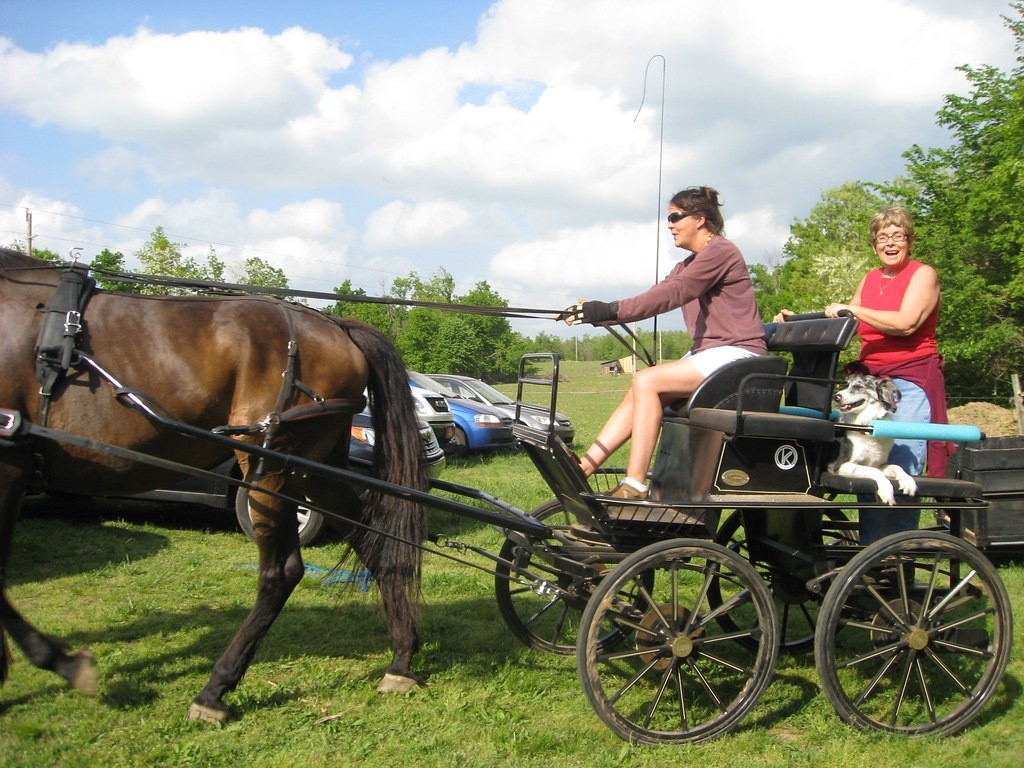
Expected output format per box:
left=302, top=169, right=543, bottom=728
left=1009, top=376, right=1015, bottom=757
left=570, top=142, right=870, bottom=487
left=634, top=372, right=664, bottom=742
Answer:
left=820, top=422, right=987, bottom=499
left=658, top=308, right=856, bottom=496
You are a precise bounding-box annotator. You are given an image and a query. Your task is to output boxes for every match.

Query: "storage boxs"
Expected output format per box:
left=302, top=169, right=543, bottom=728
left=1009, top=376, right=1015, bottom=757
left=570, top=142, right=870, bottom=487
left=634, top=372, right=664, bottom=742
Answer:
left=949, top=434, right=1024, bottom=538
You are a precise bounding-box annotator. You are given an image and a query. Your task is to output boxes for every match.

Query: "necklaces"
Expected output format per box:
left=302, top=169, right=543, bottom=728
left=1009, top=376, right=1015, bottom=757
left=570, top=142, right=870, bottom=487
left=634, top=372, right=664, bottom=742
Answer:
left=879, top=274, right=896, bottom=293
left=706, top=234, right=713, bottom=244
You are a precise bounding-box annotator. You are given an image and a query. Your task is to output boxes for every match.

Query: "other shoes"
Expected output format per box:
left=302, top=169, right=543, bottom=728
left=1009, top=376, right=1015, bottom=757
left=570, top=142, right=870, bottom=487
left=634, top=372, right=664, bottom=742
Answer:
left=596, top=478, right=649, bottom=505
left=834, top=552, right=915, bottom=593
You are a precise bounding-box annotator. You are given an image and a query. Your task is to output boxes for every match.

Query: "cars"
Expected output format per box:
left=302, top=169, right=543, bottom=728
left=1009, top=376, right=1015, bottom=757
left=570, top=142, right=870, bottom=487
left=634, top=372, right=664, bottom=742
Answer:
left=405, top=369, right=519, bottom=459
left=423, top=372, right=575, bottom=450
left=25, top=387, right=447, bottom=549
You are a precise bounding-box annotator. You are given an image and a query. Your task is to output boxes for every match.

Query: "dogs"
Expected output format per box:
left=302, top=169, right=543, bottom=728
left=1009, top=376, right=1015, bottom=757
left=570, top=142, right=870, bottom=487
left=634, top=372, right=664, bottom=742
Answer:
left=824, top=373, right=919, bottom=507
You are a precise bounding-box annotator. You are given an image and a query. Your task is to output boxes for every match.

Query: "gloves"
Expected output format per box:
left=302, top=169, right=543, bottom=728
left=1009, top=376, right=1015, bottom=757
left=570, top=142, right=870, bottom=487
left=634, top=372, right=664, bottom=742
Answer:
left=556, top=301, right=618, bottom=327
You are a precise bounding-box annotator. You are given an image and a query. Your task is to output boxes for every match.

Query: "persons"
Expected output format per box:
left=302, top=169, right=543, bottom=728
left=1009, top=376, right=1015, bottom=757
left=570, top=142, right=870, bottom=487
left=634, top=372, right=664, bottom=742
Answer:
left=562, top=186, right=766, bottom=501
left=772, top=206, right=956, bottom=586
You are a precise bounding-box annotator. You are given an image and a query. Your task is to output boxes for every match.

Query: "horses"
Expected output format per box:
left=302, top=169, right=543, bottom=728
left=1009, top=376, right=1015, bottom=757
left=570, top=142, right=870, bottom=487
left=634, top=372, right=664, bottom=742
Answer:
left=0, top=244, right=428, bottom=720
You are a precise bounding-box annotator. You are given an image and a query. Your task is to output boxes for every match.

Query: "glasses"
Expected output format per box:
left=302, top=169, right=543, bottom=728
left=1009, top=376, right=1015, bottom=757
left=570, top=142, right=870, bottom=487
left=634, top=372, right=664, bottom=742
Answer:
left=668, top=212, right=692, bottom=223
left=875, top=232, right=909, bottom=243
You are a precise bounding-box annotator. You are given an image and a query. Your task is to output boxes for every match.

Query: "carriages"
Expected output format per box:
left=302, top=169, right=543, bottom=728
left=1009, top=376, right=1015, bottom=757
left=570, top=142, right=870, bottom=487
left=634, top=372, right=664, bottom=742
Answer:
left=0, top=243, right=1015, bottom=750
